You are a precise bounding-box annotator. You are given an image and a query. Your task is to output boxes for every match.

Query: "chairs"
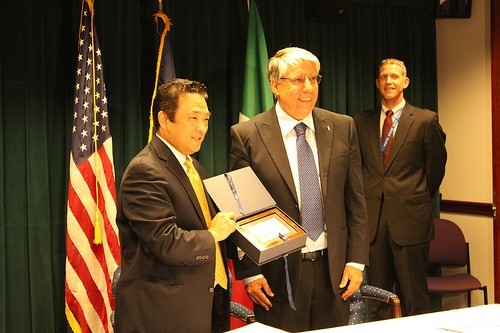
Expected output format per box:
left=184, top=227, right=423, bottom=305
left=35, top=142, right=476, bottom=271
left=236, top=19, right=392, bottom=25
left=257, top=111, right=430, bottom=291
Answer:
left=426, top=218, right=487, bottom=305
left=227, top=257, right=402, bottom=331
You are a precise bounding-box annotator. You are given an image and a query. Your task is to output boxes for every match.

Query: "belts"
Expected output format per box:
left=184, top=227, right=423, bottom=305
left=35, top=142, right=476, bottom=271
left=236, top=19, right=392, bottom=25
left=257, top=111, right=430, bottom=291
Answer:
left=301, top=248, right=327, bottom=262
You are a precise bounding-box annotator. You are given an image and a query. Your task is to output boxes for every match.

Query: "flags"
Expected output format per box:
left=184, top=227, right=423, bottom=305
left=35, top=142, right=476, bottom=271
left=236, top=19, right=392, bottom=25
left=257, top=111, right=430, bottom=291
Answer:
left=65, top=0, right=122, bottom=333
left=148, top=11, right=176, bottom=142
left=228, top=0, right=273, bottom=330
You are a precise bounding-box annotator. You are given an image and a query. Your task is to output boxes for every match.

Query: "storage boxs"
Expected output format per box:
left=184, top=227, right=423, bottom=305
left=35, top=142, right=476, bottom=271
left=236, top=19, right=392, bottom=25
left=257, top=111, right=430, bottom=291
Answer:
left=203, top=166, right=308, bottom=310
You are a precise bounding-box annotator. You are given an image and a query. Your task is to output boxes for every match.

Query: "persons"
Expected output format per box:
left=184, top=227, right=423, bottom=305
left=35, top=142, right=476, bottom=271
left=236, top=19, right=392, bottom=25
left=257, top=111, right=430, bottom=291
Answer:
left=113, top=78, right=246, bottom=333
left=353, top=57, right=448, bottom=322
left=227, top=46, right=371, bottom=333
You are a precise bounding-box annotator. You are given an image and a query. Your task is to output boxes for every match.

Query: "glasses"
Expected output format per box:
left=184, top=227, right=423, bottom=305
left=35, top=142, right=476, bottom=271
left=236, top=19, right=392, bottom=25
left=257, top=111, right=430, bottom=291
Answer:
left=280, top=73, right=323, bottom=86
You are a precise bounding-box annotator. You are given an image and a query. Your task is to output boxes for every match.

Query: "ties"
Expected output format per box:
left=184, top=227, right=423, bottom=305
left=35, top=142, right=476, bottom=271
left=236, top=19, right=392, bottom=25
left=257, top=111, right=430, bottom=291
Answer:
left=382, top=109, right=393, bottom=167
left=293, top=122, right=325, bottom=242
left=183, top=154, right=228, bottom=291
